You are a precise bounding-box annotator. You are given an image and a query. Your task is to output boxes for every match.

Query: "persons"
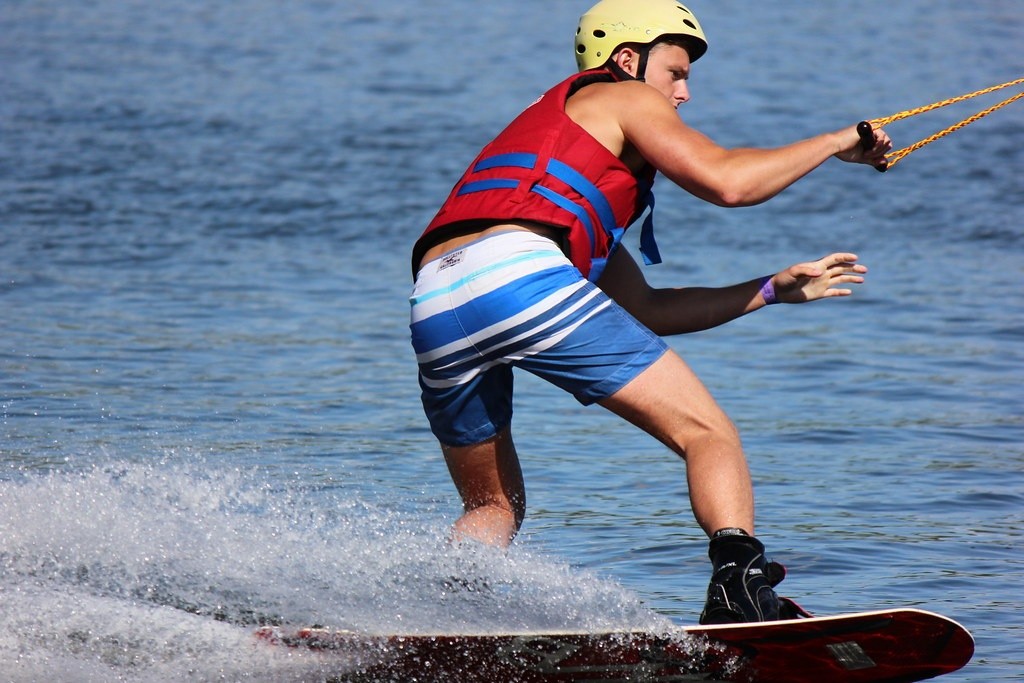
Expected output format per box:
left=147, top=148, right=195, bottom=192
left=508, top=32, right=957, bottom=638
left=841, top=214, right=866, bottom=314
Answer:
left=408, top=0, right=893, bottom=625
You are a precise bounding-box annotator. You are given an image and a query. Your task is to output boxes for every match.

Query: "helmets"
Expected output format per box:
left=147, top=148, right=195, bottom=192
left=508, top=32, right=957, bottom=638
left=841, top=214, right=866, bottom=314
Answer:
left=575, top=0, right=708, bottom=73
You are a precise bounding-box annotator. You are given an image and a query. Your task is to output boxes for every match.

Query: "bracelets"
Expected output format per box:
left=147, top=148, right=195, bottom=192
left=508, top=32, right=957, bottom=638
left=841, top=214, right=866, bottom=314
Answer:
left=760, top=276, right=779, bottom=305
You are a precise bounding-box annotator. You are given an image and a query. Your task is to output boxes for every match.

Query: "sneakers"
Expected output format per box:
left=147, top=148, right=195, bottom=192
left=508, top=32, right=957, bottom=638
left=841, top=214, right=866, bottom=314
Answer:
left=700, top=535, right=814, bottom=624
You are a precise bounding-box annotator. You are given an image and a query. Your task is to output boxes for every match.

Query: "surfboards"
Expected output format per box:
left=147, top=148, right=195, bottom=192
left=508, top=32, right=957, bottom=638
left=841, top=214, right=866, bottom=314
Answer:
left=245, top=605, right=976, bottom=683
